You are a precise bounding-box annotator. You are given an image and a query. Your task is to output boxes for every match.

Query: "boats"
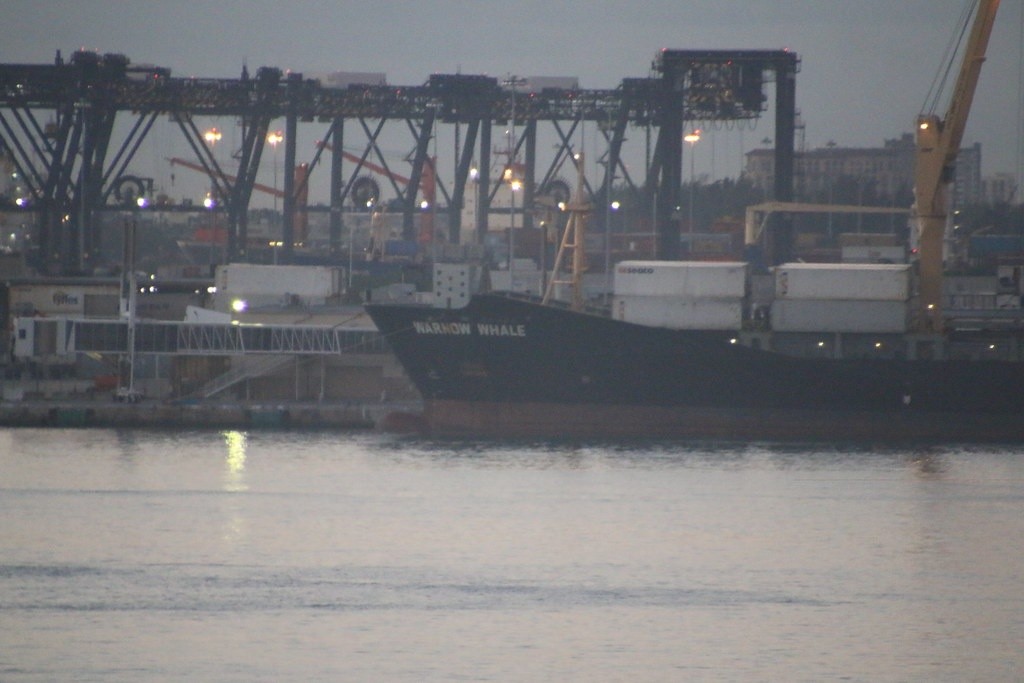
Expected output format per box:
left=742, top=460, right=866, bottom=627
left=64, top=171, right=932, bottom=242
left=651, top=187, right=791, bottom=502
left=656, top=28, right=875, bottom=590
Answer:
left=359, top=0, right=1024, bottom=441
